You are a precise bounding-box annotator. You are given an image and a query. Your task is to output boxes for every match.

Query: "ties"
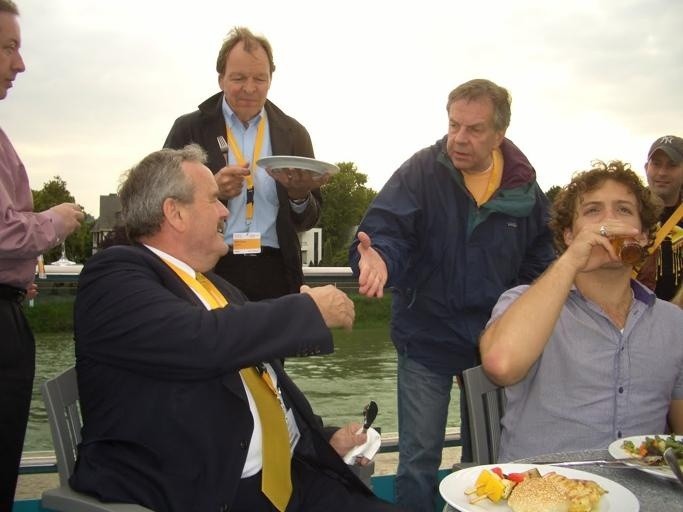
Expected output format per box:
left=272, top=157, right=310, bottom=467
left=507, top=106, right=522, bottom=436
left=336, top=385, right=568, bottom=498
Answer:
left=195, top=269, right=293, bottom=512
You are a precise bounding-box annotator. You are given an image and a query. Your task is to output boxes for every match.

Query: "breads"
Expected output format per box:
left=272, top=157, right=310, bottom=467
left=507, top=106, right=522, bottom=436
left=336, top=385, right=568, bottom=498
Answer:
left=507, top=472, right=608, bottom=512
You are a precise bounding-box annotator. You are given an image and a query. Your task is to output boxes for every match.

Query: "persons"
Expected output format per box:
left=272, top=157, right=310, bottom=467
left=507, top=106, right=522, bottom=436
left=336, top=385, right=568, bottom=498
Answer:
left=348, top=78, right=559, bottom=512
left=71, top=143, right=405, bottom=511
left=642, top=134, right=683, bottom=311
left=478, top=159, right=682, bottom=465
left=0, top=0, right=87, bottom=511
left=162, top=25, right=331, bottom=371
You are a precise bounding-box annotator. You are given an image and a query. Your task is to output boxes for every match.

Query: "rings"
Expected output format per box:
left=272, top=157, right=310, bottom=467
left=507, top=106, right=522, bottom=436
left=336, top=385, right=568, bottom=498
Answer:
left=600, top=226, right=605, bottom=236
left=288, top=174, right=293, bottom=180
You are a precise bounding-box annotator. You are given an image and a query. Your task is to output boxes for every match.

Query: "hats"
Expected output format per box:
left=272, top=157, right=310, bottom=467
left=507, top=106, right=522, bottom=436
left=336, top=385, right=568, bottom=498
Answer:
left=648, top=134, right=683, bottom=166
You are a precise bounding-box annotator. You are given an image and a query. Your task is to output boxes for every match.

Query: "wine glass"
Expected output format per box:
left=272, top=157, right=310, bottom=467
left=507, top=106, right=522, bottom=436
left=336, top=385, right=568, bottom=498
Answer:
left=50, top=206, right=84, bottom=266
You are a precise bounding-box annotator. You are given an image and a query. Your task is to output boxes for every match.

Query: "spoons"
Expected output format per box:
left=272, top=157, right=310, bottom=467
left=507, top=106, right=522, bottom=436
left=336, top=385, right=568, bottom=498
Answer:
left=360, top=399, right=377, bottom=433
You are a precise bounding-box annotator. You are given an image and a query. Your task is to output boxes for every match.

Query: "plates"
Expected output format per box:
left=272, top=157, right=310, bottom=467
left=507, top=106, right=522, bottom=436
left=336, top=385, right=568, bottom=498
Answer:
left=254, top=154, right=341, bottom=179
left=435, top=460, right=642, bottom=512
left=607, top=434, right=682, bottom=482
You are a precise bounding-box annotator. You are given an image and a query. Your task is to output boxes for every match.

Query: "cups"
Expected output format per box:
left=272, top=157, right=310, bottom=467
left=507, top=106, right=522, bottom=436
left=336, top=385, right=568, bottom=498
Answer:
left=613, top=235, right=644, bottom=265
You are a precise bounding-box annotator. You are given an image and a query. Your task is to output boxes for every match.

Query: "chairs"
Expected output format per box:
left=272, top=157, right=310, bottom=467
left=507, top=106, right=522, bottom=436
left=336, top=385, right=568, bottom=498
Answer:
left=43, top=365, right=160, bottom=512
left=453, top=365, right=506, bottom=471
